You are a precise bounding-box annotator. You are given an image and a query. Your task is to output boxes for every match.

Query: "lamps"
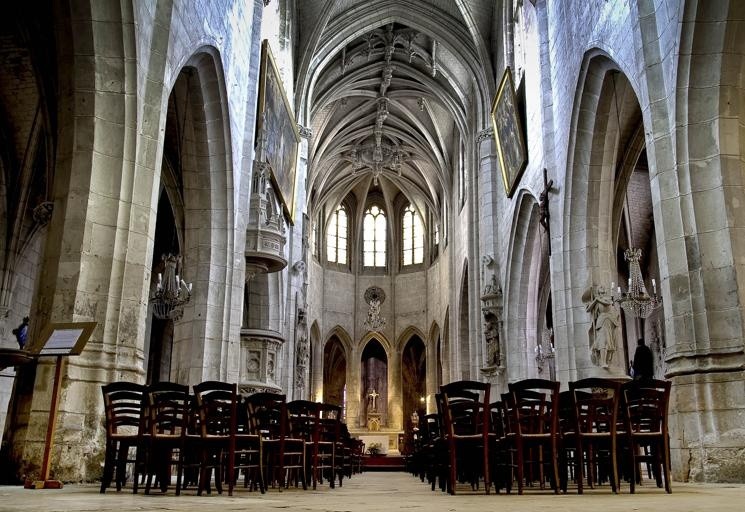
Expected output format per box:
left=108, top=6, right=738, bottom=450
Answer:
left=534, top=344, right=556, bottom=373
left=609, top=74, right=663, bottom=320
left=148, top=226, right=193, bottom=318
left=362, top=299, right=387, bottom=331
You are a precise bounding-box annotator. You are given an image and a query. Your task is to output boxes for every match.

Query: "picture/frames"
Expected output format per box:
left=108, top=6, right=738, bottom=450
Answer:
left=490, top=65, right=528, bottom=199
left=256, top=39, right=300, bottom=226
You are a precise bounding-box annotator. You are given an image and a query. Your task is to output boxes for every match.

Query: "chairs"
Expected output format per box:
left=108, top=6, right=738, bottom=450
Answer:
left=405, top=380, right=672, bottom=495
left=101, top=379, right=365, bottom=496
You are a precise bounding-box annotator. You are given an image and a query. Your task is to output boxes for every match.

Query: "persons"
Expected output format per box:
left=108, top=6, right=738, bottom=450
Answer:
left=587, top=284, right=619, bottom=369
left=630, top=337, right=654, bottom=379
left=13, top=317, right=29, bottom=370
left=297, top=334, right=308, bottom=387
